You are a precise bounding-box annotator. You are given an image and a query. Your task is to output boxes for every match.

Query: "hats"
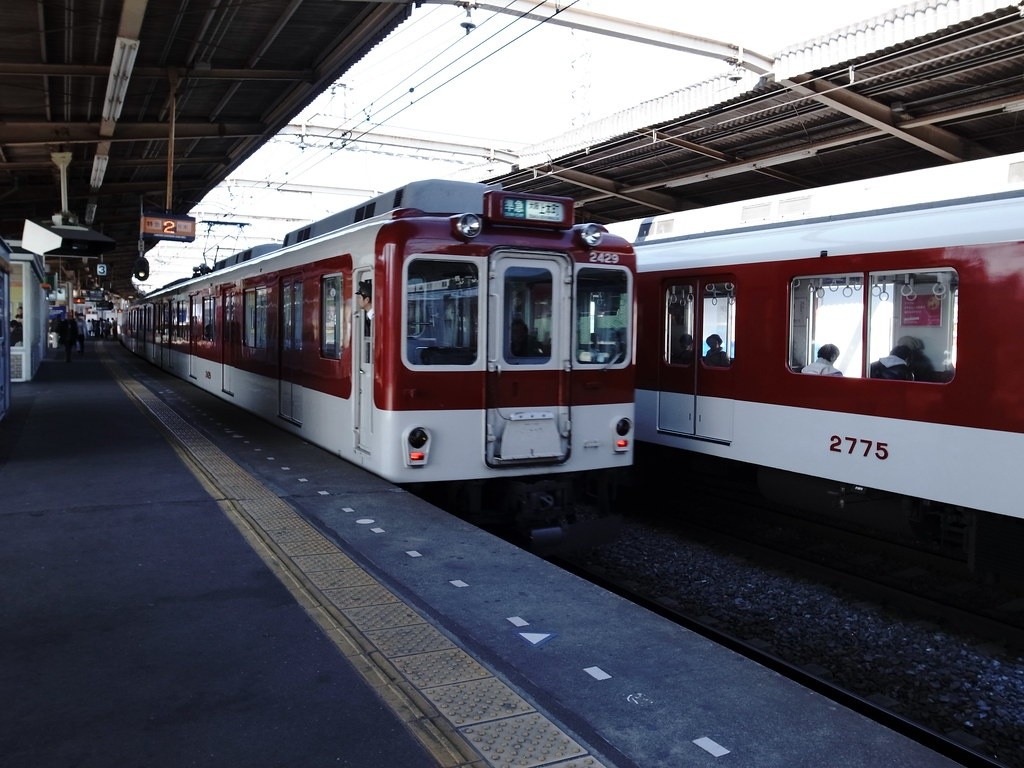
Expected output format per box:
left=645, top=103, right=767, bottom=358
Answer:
left=706, top=334, right=722, bottom=348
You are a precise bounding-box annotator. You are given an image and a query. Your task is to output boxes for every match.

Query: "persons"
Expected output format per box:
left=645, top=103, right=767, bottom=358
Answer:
left=510, top=319, right=537, bottom=359
left=870, top=335, right=936, bottom=382
left=705, top=334, right=726, bottom=364
left=9, top=306, right=23, bottom=346
left=671, top=334, right=693, bottom=364
left=800, top=344, right=843, bottom=376
left=87, top=318, right=117, bottom=340
left=355, top=280, right=373, bottom=335
left=50, top=309, right=88, bottom=361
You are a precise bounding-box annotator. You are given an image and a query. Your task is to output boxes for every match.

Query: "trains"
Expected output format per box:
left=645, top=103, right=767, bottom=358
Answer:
left=116, top=180, right=639, bottom=486
left=535, top=190, right=1024, bottom=563
